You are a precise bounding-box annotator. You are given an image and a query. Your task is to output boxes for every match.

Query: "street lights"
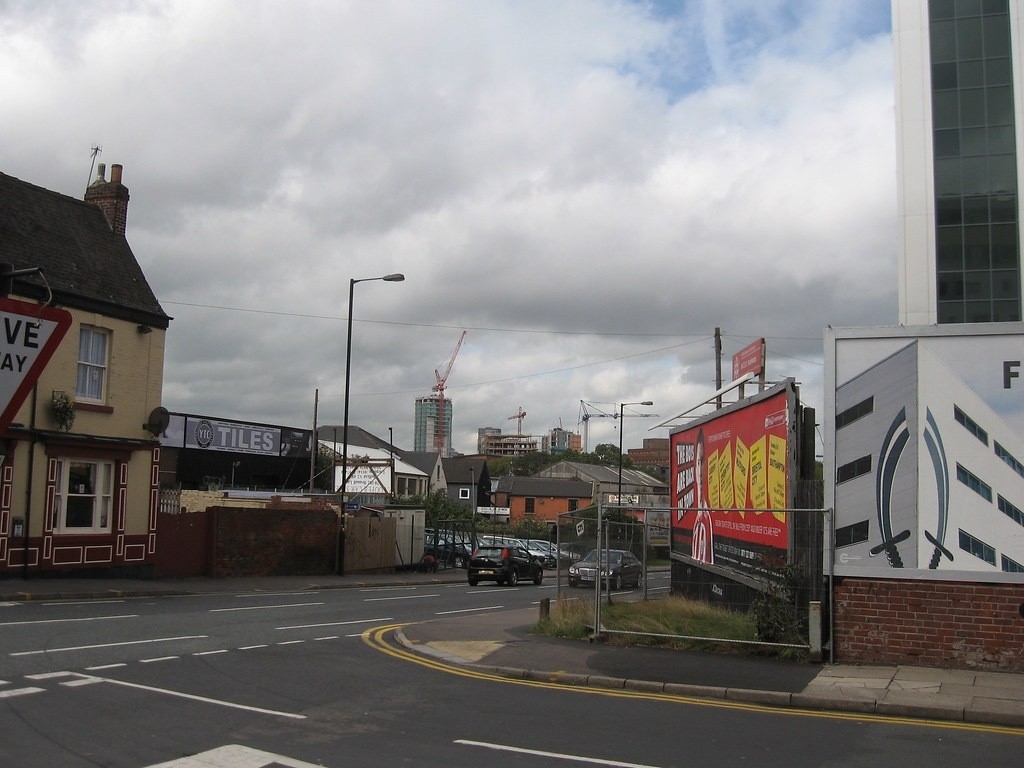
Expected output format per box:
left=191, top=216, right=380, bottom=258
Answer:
left=618, top=401, right=654, bottom=541
left=338, top=273, right=406, bottom=576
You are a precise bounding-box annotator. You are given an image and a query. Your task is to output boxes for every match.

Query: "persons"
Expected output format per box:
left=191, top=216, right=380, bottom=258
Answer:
left=691, top=429, right=714, bottom=564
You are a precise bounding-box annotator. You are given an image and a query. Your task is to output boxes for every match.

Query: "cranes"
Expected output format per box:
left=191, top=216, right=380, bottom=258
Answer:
left=507, top=407, right=526, bottom=442
left=576, top=400, right=661, bottom=456
left=430, top=329, right=467, bottom=458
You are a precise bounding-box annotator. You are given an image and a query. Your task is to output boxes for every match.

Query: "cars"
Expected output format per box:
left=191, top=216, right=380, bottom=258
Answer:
left=568, top=548, right=643, bottom=592
left=419, top=530, right=584, bottom=572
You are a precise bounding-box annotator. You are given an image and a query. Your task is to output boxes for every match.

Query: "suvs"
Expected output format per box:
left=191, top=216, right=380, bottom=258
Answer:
left=467, top=543, right=544, bottom=587
